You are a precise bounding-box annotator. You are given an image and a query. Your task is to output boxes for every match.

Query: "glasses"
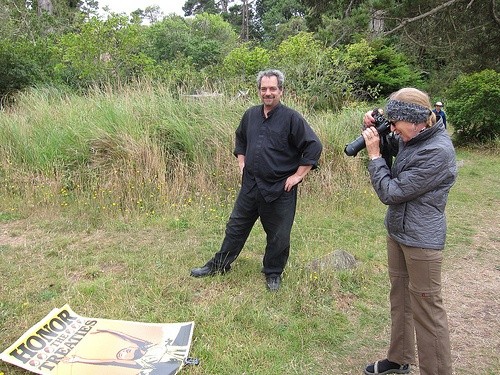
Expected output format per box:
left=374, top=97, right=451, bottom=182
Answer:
left=389, top=120, right=400, bottom=127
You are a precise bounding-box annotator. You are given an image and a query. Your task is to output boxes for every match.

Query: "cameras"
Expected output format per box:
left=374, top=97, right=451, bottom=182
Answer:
left=344, top=112, right=392, bottom=158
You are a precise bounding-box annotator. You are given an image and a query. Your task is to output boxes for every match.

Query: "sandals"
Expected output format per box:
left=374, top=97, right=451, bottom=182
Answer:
left=365, top=360, right=411, bottom=375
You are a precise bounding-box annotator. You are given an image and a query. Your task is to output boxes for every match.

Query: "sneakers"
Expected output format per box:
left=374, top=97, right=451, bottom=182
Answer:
left=266, top=276, right=280, bottom=290
left=191, top=266, right=211, bottom=276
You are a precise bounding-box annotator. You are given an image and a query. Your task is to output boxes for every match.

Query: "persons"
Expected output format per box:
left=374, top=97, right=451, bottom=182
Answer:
left=190, top=69, right=322, bottom=291
left=359, top=87, right=456, bottom=375
left=432, top=102, right=447, bottom=130
left=62, top=328, right=187, bottom=372
